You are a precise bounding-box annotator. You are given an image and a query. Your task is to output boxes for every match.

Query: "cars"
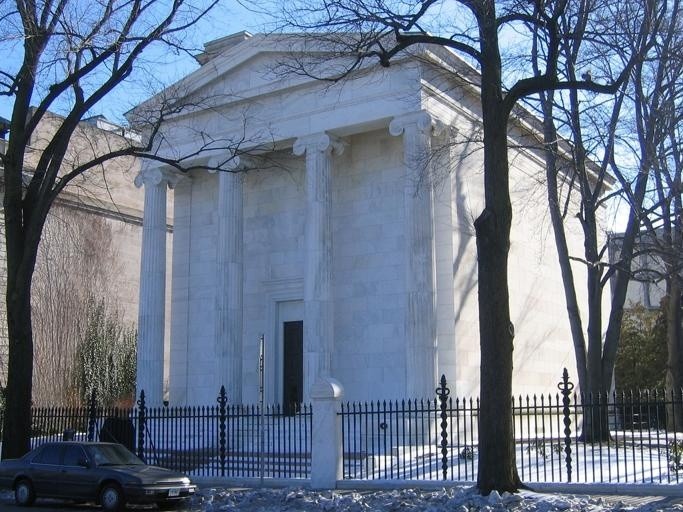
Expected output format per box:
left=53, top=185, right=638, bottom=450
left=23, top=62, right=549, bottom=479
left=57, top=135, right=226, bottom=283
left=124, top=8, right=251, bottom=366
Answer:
left=0, top=440, right=197, bottom=509
